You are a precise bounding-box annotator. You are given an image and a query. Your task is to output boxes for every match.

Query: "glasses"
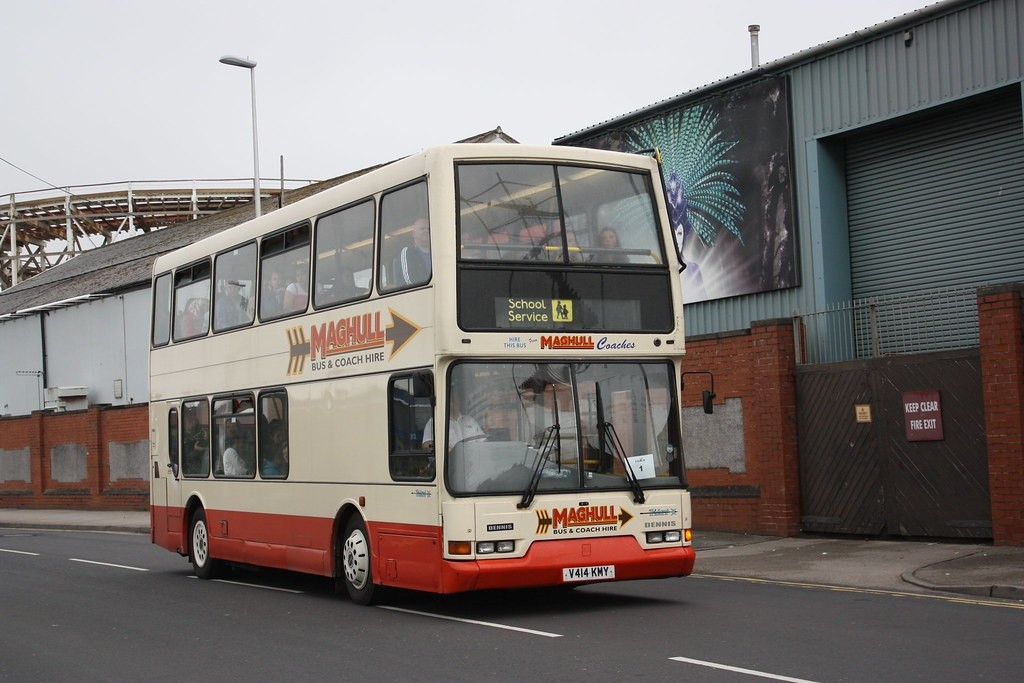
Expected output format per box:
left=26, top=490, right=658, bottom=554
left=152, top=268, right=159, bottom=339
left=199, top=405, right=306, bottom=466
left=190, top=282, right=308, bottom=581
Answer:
left=522, top=394, right=535, bottom=401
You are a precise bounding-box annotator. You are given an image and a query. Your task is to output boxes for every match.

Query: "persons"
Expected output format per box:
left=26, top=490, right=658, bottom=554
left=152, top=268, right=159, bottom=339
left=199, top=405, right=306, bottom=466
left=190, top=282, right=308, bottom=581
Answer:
left=585, top=228, right=631, bottom=263
left=223, top=428, right=256, bottom=476
left=216, top=278, right=247, bottom=331
left=186, top=427, right=209, bottom=474
left=321, top=267, right=368, bottom=306
left=263, top=263, right=309, bottom=318
left=422, top=387, right=488, bottom=468
left=176, top=296, right=209, bottom=339
left=397, top=217, right=431, bottom=287
left=260, top=418, right=289, bottom=476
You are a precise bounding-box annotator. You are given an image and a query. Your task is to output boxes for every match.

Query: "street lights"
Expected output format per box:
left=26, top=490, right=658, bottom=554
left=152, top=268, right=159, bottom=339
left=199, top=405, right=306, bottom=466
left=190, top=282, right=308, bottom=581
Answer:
left=219, top=54, right=262, bottom=218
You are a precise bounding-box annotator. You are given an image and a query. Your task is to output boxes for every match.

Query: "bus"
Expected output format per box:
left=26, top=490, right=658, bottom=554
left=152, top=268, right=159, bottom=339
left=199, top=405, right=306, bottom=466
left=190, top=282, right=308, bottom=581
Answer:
left=148, top=143, right=717, bottom=606
